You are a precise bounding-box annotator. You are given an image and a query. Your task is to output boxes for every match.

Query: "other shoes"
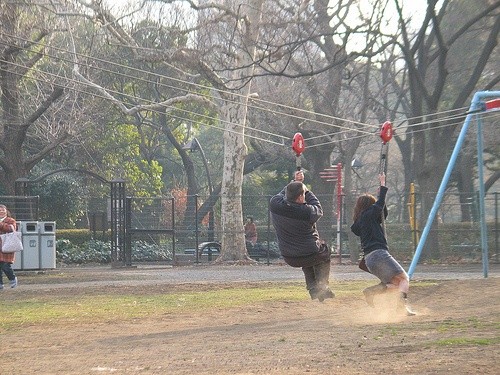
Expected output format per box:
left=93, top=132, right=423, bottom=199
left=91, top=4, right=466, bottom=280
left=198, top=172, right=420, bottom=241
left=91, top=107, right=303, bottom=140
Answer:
left=317, top=288, right=335, bottom=298
left=0, top=284, right=4, bottom=289
left=9, top=277, right=17, bottom=288
left=311, top=293, right=324, bottom=301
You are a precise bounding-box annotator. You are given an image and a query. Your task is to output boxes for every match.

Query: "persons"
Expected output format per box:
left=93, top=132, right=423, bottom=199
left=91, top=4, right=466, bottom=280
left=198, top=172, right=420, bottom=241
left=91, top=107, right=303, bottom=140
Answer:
left=269, top=170, right=337, bottom=303
left=243, top=215, right=258, bottom=245
left=0, top=204, right=24, bottom=290
left=350, top=172, right=417, bottom=317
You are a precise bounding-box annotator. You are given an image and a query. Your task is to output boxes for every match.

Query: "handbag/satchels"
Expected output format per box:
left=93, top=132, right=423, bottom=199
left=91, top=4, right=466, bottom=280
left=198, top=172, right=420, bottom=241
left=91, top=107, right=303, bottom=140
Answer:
left=359, top=257, right=369, bottom=272
left=0, top=223, right=24, bottom=253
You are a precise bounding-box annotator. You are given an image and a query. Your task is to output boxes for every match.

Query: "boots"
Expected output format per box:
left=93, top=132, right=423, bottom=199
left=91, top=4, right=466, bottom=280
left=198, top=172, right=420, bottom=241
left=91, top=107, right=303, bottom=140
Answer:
left=396, top=292, right=417, bottom=316
left=363, top=282, right=388, bottom=306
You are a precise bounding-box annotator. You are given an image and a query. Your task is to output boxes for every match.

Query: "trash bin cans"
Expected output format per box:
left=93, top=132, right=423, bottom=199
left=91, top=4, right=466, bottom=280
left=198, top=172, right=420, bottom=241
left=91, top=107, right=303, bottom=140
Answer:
left=22, top=221, right=39, bottom=270
left=40, top=221, right=56, bottom=269
left=10, top=221, right=21, bottom=270
